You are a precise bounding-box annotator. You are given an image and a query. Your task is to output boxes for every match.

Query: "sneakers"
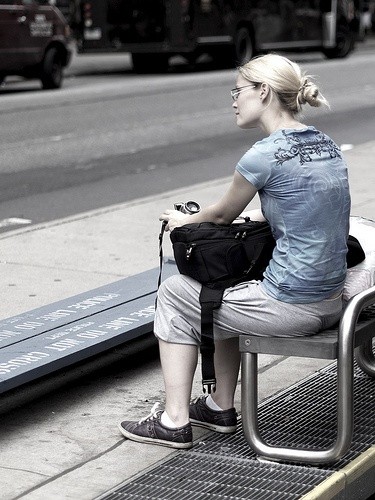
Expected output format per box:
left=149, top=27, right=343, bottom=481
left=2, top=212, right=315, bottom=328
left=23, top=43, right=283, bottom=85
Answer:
left=188, top=395, right=237, bottom=433
left=119, top=410, right=193, bottom=448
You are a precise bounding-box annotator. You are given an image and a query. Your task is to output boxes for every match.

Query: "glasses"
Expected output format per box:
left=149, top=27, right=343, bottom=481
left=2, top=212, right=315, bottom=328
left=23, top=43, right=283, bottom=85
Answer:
left=230, top=83, right=261, bottom=101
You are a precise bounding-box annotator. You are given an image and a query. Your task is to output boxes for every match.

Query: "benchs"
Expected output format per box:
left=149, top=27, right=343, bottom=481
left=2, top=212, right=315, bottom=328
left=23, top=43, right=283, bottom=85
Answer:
left=238, top=286, right=375, bottom=464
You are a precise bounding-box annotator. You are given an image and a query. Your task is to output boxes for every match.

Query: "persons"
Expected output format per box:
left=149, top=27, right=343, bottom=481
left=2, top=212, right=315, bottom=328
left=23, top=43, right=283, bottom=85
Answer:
left=119, top=54, right=351, bottom=450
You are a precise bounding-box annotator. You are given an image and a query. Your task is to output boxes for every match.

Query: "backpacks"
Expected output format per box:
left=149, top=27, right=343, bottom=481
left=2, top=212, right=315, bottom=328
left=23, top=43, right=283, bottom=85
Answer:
left=170, top=222, right=365, bottom=394
